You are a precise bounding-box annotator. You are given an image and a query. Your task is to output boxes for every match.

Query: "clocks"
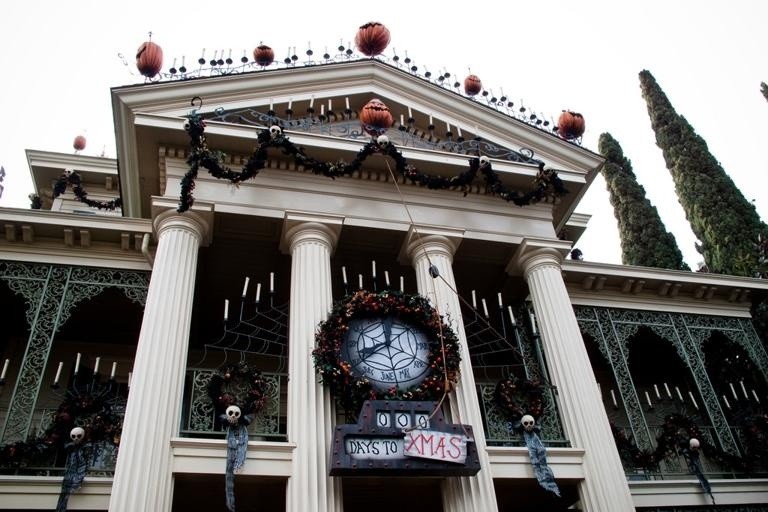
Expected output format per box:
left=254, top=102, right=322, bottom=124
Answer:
left=339, top=311, right=434, bottom=394
